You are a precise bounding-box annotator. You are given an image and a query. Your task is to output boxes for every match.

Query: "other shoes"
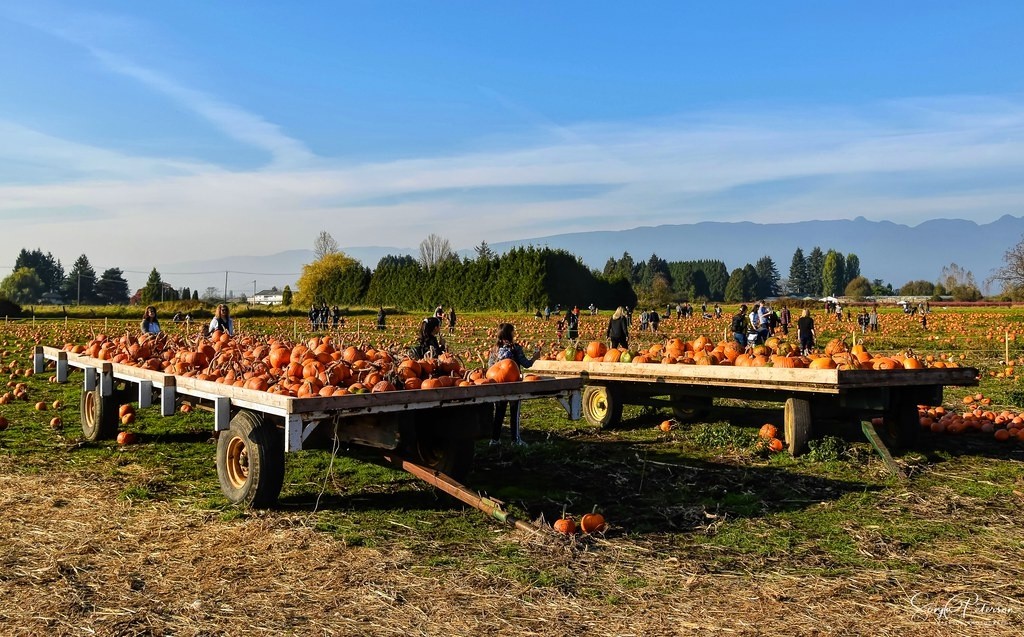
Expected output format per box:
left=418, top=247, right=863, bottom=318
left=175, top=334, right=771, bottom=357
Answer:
left=489, top=440, right=502, bottom=446
left=511, top=441, right=527, bottom=446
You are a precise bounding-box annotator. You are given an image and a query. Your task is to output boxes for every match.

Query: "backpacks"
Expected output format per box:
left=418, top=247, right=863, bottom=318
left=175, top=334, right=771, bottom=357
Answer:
left=731, top=314, right=748, bottom=333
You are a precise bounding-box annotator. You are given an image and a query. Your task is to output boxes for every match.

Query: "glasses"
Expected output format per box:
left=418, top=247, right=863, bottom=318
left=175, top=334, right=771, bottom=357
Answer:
left=221, top=309, right=228, bottom=311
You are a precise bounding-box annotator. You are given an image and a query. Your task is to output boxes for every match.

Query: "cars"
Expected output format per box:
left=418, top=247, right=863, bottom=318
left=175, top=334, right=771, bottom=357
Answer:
left=897, top=301, right=910, bottom=306
left=819, top=296, right=837, bottom=303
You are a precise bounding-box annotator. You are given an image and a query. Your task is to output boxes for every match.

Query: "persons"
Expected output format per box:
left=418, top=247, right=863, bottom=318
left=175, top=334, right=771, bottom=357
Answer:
left=308, top=301, right=345, bottom=331
left=418, top=317, right=448, bottom=360
left=488, top=323, right=540, bottom=446
left=447, top=308, right=457, bottom=334
left=377, top=306, right=386, bottom=330
left=208, top=304, right=233, bottom=336
left=172, top=311, right=193, bottom=322
left=433, top=304, right=444, bottom=327
left=535, top=294, right=930, bottom=356
left=140, top=305, right=161, bottom=335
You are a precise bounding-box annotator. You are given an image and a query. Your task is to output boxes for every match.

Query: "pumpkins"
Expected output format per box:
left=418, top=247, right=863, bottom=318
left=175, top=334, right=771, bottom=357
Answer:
left=455, top=313, right=734, bottom=364
left=759, top=423, right=783, bottom=452
left=872, top=392, right=1024, bottom=442
left=0, top=316, right=542, bottom=412
left=555, top=333, right=961, bottom=369
left=0, top=417, right=8, bottom=431
left=769, top=311, right=1024, bottom=381
left=50, top=418, right=63, bottom=429
left=119, top=403, right=135, bottom=419
left=554, top=505, right=575, bottom=535
left=659, top=420, right=671, bottom=431
left=121, top=413, right=136, bottom=425
left=580, top=504, right=605, bottom=534
left=117, top=431, right=136, bottom=444
